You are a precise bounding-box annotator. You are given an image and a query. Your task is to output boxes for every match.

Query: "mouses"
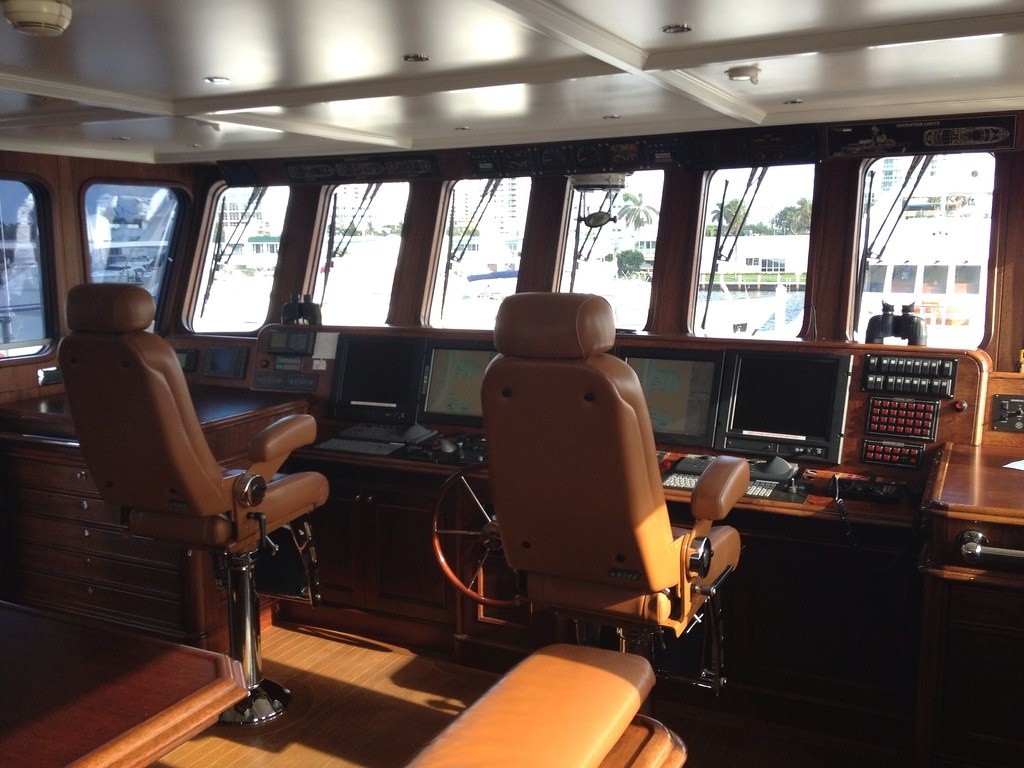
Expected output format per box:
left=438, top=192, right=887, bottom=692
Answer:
left=421, top=437, right=456, bottom=453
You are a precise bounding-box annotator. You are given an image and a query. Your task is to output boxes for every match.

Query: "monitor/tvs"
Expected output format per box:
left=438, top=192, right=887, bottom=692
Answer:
left=418, top=338, right=503, bottom=427
left=713, top=348, right=854, bottom=465
left=615, top=346, right=725, bottom=449
left=325, top=334, right=429, bottom=425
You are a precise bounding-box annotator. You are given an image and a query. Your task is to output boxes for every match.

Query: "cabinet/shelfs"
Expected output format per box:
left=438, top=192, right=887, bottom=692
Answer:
left=917, top=442, right=1024, bottom=768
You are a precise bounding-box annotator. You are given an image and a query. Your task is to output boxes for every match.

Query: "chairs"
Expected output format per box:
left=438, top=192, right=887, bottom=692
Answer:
left=55, top=284, right=322, bottom=728
left=480, top=293, right=751, bottom=727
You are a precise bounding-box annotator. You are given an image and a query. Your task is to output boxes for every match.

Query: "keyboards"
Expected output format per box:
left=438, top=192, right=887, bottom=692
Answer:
left=314, top=438, right=406, bottom=456
left=662, top=472, right=780, bottom=498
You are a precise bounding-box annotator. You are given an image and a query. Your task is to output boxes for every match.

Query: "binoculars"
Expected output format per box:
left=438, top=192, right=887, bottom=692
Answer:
left=280, top=294, right=323, bottom=328
left=863, top=300, right=927, bottom=345
